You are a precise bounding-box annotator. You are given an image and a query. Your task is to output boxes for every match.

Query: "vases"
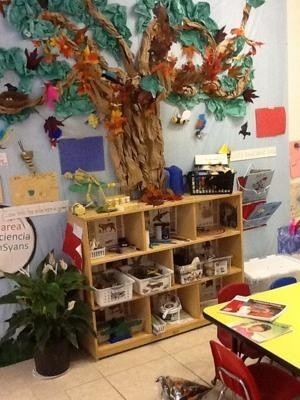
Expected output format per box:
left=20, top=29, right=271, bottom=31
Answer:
left=32, top=340, right=70, bottom=376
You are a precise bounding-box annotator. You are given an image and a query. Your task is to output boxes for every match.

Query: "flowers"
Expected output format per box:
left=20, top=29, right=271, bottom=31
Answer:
left=0, top=251, right=98, bottom=351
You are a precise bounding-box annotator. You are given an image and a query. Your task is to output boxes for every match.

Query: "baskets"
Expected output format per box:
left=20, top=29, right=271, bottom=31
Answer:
left=172, top=246, right=205, bottom=285
left=117, top=256, right=174, bottom=297
left=88, top=265, right=136, bottom=308
left=194, top=240, right=236, bottom=279
left=186, top=168, right=237, bottom=196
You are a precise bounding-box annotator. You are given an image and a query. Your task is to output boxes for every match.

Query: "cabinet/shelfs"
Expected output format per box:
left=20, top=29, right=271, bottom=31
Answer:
left=67, top=195, right=245, bottom=360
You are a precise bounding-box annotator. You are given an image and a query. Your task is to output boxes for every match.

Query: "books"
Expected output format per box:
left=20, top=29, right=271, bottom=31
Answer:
left=241, top=167, right=282, bottom=228
left=221, top=294, right=287, bottom=324
left=230, top=319, right=294, bottom=344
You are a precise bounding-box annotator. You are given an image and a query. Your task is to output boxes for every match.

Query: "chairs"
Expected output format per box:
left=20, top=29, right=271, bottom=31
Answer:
left=202, top=275, right=300, bottom=400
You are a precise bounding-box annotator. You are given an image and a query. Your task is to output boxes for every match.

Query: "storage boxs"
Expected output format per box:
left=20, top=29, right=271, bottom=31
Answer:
left=244, top=254, right=300, bottom=293
